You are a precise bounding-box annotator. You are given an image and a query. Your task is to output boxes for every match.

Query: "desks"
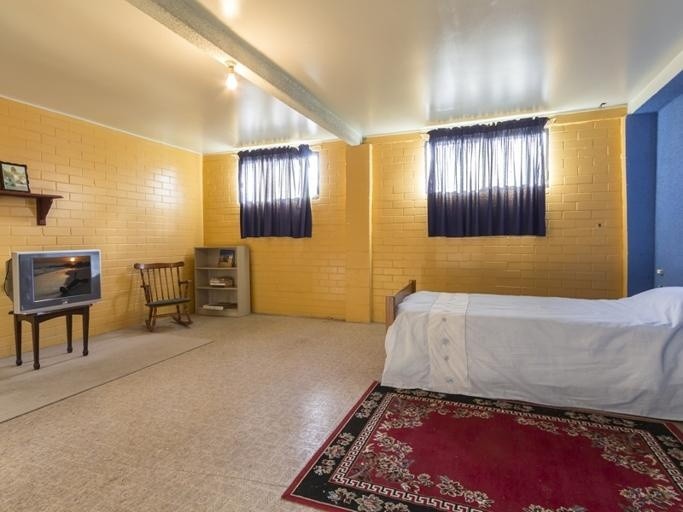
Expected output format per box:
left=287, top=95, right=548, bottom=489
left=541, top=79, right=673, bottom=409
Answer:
left=7, top=303, right=93, bottom=370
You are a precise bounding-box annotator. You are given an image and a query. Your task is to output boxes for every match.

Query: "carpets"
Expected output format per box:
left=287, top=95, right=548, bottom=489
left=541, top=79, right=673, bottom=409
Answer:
left=280, top=380, right=683, bottom=512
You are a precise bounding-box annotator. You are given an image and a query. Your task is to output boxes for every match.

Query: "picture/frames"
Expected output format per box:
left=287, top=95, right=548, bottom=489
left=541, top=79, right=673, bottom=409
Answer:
left=216, top=249, right=235, bottom=267
left=0, top=161, right=31, bottom=193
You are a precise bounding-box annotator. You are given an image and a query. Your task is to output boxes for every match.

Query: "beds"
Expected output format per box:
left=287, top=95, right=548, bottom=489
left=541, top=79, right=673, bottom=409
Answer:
left=380, top=279, right=683, bottom=422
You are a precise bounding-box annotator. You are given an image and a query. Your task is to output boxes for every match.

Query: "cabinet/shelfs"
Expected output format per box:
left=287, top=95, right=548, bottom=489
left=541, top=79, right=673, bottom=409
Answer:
left=193, top=246, right=251, bottom=317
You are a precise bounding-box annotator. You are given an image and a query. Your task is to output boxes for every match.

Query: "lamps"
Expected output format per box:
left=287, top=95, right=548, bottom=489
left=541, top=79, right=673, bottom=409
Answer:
left=225, top=60, right=238, bottom=91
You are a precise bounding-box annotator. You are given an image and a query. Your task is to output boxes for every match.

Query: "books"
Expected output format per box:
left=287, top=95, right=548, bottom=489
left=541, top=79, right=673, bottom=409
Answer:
left=203, top=303, right=237, bottom=310
left=209, top=276, right=235, bottom=287
left=218, top=250, right=233, bottom=267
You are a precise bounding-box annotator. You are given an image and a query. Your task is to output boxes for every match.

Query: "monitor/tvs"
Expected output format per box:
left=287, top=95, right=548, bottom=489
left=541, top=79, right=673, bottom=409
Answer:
left=12, top=249, right=101, bottom=316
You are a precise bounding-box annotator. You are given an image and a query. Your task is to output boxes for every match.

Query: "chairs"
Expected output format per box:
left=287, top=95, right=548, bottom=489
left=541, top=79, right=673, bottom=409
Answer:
left=134, top=262, right=193, bottom=332
